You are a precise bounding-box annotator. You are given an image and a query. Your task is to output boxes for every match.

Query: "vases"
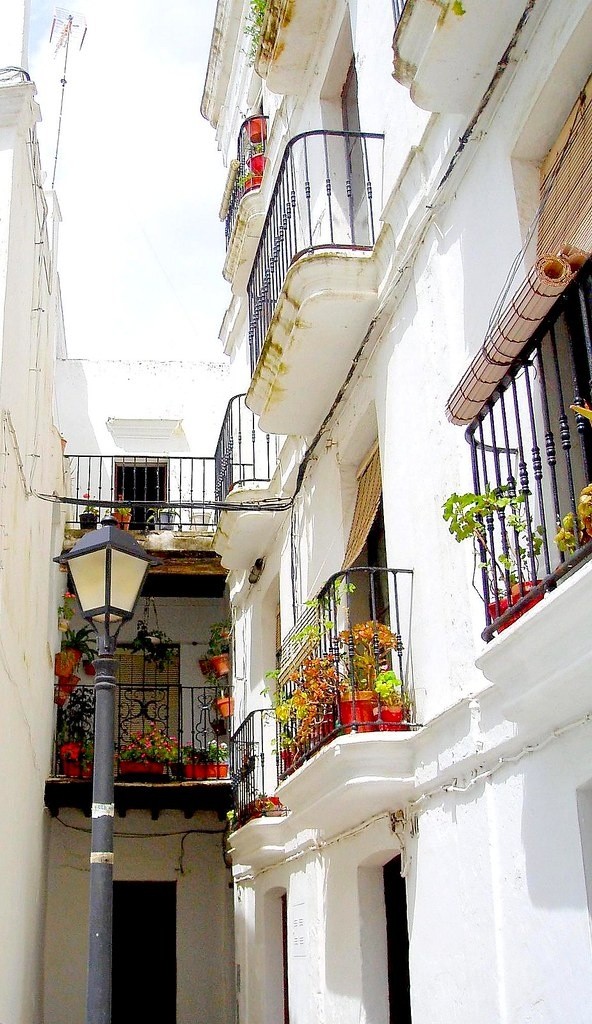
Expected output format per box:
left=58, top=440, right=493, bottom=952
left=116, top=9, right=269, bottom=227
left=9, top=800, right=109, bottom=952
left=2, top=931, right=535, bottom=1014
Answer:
left=189, top=511, right=213, bottom=533
left=118, top=759, right=166, bottom=778
left=79, top=513, right=97, bottom=530
left=61, top=759, right=94, bottom=778
left=172, top=762, right=230, bottom=778
left=58, top=619, right=70, bottom=633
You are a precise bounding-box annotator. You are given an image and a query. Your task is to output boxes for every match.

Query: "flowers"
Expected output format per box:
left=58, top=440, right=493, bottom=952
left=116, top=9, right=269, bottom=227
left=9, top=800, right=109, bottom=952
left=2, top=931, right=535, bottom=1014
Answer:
left=59, top=740, right=94, bottom=773
left=114, top=722, right=180, bottom=765
left=58, top=592, right=76, bottom=621
left=82, top=493, right=98, bottom=515
left=180, top=740, right=231, bottom=764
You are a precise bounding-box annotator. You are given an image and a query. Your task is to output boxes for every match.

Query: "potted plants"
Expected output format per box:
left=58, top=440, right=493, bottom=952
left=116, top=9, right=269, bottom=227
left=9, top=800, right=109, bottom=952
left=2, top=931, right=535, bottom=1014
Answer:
left=61, top=432, right=67, bottom=454
left=53, top=623, right=98, bottom=707
left=552, top=482, right=592, bottom=564
left=146, top=506, right=181, bottom=530
left=198, top=618, right=234, bottom=735
left=242, top=114, right=267, bottom=194
left=108, top=494, right=133, bottom=532
left=442, top=480, right=547, bottom=636
left=234, top=796, right=283, bottom=832
left=130, top=596, right=176, bottom=673
left=276, top=620, right=414, bottom=777
